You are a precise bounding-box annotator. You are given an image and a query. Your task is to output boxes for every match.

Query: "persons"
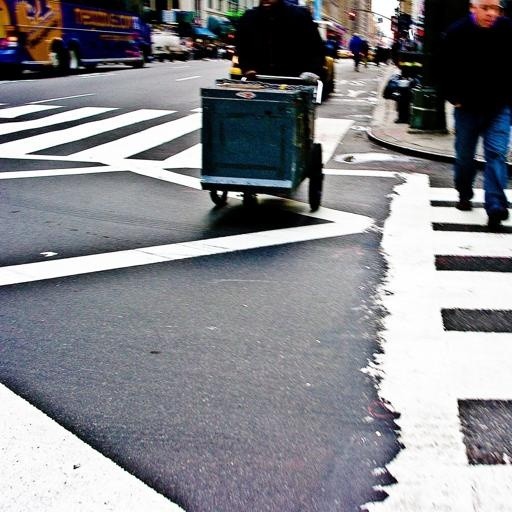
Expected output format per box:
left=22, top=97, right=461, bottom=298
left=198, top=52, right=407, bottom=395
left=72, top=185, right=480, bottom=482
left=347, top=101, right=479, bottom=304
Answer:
left=363, top=39, right=370, bottom=69
left=325, top=34, right=345, bottom=55
left=439, top=0, right=512, bottom=227
left=348, top=33, right=363, bottom=71
left=235, top=0, right=335, bottom=103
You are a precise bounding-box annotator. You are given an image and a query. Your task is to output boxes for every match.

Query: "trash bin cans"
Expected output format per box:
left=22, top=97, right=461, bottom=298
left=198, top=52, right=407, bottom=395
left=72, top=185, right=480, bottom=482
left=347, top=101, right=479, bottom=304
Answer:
left=412, top=86, right=446, bottom=133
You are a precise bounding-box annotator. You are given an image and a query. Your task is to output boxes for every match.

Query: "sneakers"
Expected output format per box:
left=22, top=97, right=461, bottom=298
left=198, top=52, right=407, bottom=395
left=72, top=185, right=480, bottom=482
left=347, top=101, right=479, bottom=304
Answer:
left=456, top=176, right=473, bottom=201
left=488, top=208, right=508, bottom=225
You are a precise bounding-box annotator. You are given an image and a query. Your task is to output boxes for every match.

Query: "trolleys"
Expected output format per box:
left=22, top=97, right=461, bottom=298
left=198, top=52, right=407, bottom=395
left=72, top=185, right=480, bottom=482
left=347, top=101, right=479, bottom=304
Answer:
left=199, top=68, right=328, bottom=213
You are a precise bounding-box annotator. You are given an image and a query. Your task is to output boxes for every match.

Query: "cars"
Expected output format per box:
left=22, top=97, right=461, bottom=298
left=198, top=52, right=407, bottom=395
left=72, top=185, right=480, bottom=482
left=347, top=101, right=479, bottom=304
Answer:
left=0, top=34, right=23, bottom=77
left=337, top=46, right=378, bottom=63
left=150, top=26, right=235, bottom=62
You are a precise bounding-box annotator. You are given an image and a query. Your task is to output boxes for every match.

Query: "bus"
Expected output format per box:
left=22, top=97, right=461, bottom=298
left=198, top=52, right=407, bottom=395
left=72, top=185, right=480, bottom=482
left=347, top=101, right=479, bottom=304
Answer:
left=0, top=0, right=153, bottom=73
left=314, top=21, right=348, bottom=48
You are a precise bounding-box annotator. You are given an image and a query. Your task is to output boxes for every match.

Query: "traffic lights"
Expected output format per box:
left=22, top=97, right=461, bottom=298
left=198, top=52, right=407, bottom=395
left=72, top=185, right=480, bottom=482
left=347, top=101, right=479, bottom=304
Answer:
left=350, top=10, right=355, bottom=21
left=399, top=15, right=413, bottom=37
